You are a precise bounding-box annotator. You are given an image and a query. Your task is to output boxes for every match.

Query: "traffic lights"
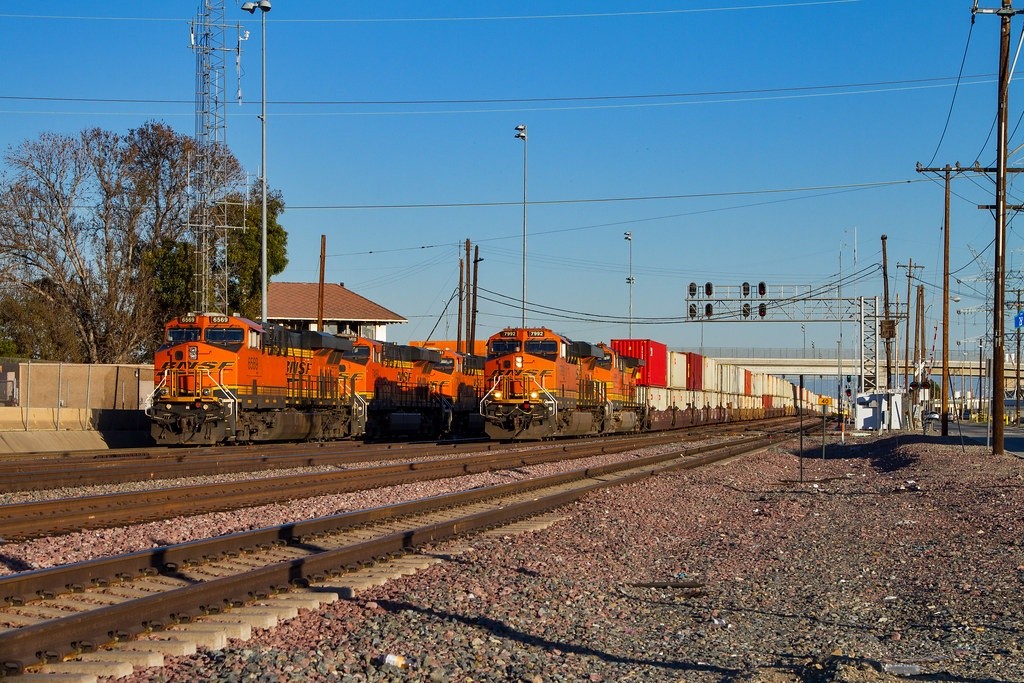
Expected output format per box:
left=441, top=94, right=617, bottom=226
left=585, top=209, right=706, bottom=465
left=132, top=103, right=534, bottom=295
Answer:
left=922, top=382, right=930, bottom=388
left=704, top=283, right=713, bottom=298
left=757, top=281, right=768, bottom=297
left=758, top=305, right=767, bottom=318
left=911, top=382, right=918, bottom=389
left=705, top=303, right=713, bottom=317
left=846, top=388, right=852, bottom=396
left=847, top=374, right=852, bottom=383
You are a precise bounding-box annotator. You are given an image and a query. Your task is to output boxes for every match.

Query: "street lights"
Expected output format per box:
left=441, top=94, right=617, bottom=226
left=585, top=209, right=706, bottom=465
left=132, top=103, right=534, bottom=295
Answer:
left=512, top=124, right=528, bottom=328
left=236, top=0, right=273, bottom=328
left=623, top=229, right=633, bottom=336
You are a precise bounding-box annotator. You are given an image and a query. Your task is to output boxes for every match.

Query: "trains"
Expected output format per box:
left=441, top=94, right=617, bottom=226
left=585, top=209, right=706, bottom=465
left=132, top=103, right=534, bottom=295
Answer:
left=479, top=326, right=854, bottom=447
left=147, top=310, right=490, bottom=444
